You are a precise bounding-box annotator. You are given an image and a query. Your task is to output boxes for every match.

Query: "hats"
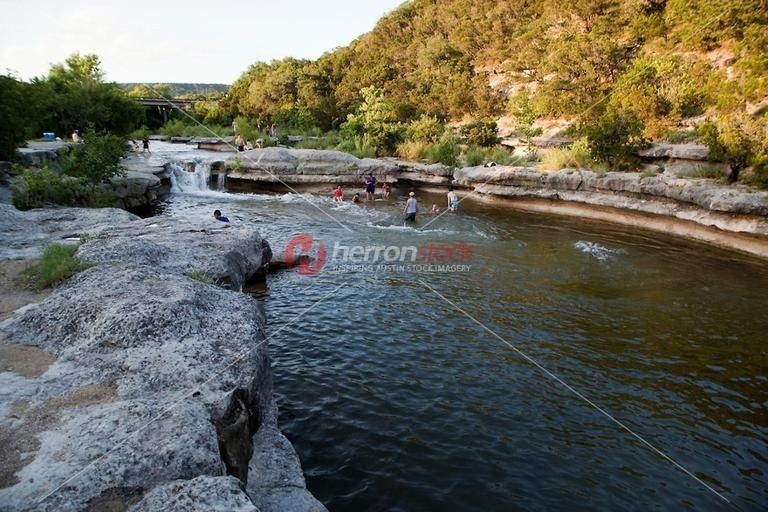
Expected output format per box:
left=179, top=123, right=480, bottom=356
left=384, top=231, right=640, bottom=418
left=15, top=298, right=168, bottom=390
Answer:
left=409, top=192, right=415, bottom=195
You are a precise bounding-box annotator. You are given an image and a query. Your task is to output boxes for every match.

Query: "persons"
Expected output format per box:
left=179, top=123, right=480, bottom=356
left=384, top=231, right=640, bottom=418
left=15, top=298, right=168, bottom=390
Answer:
left=231, top=116, right=277, bottom=152
left=333, top=185, right=343, bottom=202
left=381, top=182, right=391, bottom=200
left=131, top=139, right=139, bottom=151
left=214, top=209, right=229, bottom=222
left=363, top=173, right=377, bottom=201
left=446, top=188, right=458, bottom=211
left=140, top=136, right=151, bottom=152
left=71, top=130, right=82, bottom=143
left=351, top=193, right=360, bottom=203
left=402, top=192, right=418, bottom=227
